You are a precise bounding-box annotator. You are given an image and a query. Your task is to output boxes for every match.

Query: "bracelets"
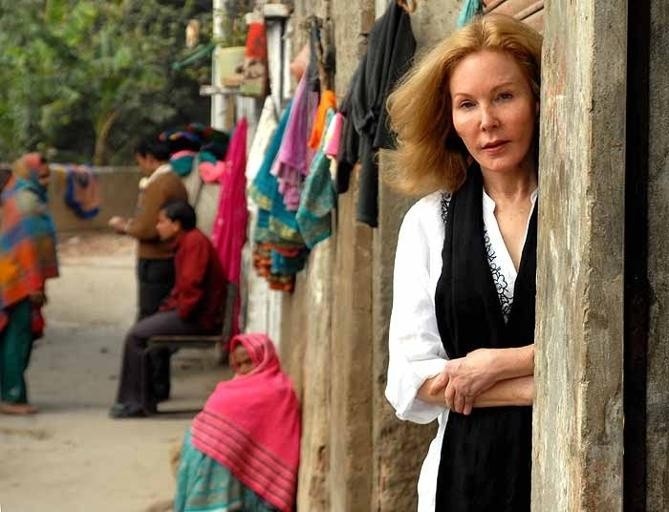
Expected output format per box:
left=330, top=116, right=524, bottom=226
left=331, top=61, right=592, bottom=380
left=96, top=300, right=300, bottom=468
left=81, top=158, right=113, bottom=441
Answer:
left=124, top=224, right=128, bottom=232
left=127, top=218, right=132, bottom=225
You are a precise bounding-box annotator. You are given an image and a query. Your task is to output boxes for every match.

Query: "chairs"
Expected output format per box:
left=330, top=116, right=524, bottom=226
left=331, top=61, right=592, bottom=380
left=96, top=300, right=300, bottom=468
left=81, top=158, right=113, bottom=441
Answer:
left=140, top=283, right=236, bottom=413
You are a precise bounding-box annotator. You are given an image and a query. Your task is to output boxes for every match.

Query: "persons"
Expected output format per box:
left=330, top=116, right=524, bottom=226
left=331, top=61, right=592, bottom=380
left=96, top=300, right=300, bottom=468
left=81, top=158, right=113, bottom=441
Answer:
left=109, top=196, right=225, bottom=418
left=0, top=153, right=57, bottom=415
left=371, top=15, right=549, bottom=512
left=143, top=332, right=302, bottom=510
left=107, top=139, right=189, bottom=316
